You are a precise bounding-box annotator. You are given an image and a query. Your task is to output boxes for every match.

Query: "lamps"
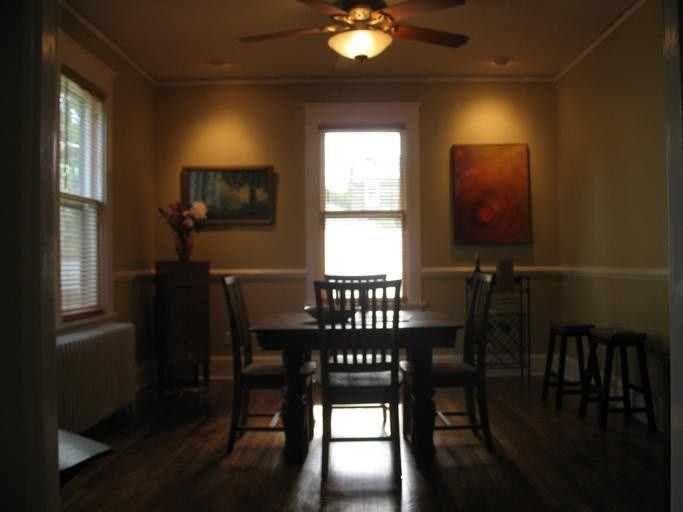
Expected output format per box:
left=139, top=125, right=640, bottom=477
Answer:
left=326, top=29, right=394, bottom=61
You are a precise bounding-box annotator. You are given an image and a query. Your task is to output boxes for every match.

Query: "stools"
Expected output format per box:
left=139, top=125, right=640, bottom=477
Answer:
left=541, top=321, right=599, bottom=411
left=580, top=327, right=655, bottom=432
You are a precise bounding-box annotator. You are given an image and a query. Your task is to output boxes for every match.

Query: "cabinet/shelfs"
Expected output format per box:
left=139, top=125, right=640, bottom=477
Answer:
left=158, top=256, right=211, bottom=390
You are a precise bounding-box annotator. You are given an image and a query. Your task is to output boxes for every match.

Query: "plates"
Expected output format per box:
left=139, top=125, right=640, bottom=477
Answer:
left=303, top=304, right=360, bottom=321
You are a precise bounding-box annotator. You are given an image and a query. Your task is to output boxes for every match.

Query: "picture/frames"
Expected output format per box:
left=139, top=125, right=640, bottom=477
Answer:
left=180, top=166, right=274, bottom=226
left=451, top=143, right=533, bottom=246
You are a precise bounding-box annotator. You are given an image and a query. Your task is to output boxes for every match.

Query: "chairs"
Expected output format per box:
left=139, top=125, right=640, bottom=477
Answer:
left=314, top=280, right=401, bottom=451
left=222, top=275, right=316, bottom=455
left=399, top=272, right=497, bottom=453
left=324, top=274, right=392, bottom=365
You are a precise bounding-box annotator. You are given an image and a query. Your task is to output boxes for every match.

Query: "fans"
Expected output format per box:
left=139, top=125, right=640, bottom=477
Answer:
left=240, top=0, right=468, bottom=49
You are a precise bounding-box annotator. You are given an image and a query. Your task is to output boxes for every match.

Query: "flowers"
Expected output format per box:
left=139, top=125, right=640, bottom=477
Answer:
left=158, top=200, right=207, bottom=233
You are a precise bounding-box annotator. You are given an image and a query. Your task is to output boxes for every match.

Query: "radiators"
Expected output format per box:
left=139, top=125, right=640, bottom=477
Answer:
left=56, top=324, right=137, bottom=436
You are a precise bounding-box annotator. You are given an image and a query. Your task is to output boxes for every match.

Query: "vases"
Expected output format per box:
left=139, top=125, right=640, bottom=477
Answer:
left=175, top=233, right=193, bottom=261
